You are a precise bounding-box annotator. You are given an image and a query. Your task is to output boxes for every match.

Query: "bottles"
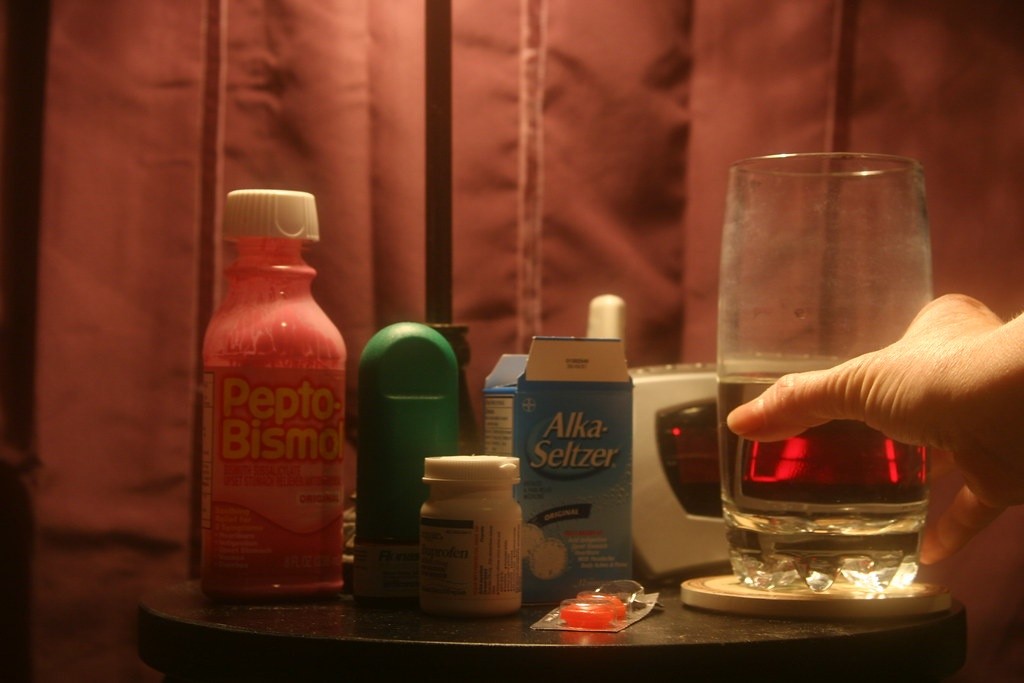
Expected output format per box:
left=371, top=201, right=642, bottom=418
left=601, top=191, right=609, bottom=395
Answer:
left=422, top=453, right=524, bottom=615
left=200, top=187, right=347, bottom=598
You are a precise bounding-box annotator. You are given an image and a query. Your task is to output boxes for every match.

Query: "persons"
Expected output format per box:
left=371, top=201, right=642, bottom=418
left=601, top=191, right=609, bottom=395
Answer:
left=726, top=292, right=1024, bottom=566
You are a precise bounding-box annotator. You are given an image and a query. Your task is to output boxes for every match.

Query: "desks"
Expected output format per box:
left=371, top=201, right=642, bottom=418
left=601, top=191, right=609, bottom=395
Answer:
left=136, top=579, right=969, bottom=683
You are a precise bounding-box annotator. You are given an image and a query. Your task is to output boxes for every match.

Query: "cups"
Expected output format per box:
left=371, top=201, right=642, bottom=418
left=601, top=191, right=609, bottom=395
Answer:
left=716, top=152, right=935, bottom=595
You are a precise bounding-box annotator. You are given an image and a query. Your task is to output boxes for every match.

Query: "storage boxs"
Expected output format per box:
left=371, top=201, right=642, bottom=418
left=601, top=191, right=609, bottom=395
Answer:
left=482, top=337, right=633, bottom=608
